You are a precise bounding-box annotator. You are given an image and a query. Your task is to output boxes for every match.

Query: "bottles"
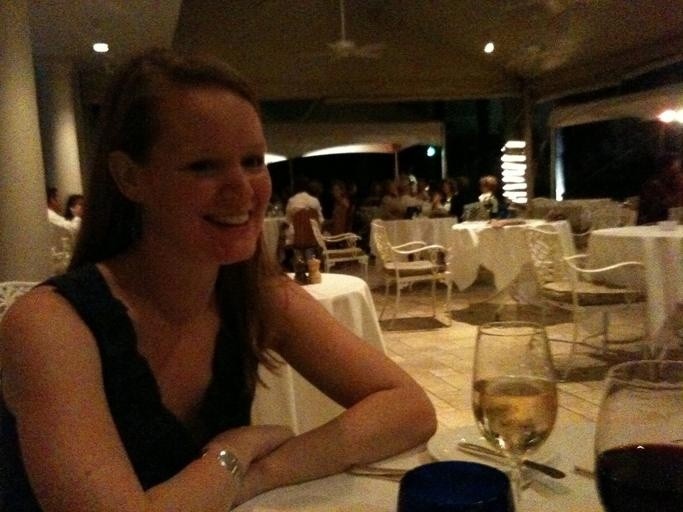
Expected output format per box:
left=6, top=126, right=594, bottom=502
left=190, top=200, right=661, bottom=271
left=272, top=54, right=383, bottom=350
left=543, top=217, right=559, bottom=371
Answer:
left=293, top=254, right=321, bottom=285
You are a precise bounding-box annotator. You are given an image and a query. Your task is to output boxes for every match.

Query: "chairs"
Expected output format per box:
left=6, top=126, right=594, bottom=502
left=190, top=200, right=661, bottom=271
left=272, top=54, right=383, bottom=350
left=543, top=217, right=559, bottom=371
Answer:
left=310, top=220, right=371, bottom=277
left=372, top=218, right=455, bottom=330
left=523, top=226, right=652, bottom=381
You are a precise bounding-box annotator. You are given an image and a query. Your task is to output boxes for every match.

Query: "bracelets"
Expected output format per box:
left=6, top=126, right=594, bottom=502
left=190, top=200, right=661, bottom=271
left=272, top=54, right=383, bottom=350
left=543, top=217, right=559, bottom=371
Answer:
left=204, top=444, right=246, bottom=490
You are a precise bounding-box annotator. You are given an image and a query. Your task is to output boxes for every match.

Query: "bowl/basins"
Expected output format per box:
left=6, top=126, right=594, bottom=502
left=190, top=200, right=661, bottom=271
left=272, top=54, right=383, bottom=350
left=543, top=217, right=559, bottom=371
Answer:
left=657, top=221, right=677, bottom=231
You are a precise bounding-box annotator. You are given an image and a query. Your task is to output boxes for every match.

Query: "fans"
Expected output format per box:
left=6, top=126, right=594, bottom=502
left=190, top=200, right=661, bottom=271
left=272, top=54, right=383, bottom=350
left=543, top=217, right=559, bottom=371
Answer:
left=295, top=1, right=384, bottom=66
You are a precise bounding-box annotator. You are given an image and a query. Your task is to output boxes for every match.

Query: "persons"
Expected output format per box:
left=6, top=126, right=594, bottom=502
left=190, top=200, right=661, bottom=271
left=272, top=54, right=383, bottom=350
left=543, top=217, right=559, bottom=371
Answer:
left=377, top=170, right=469, bottom=223
left=0, top=45, right=438, bottom=512
left=634, top=154, right=683, bottom=226
left=64, top=193, right=86, bottom=227
left=283, top=179, right=327, bottom=270
left=476, top=175, right=499, bottom=218
left=320, top=182, right=349, bottom=235
left=43, top=185, right=72, bottom=232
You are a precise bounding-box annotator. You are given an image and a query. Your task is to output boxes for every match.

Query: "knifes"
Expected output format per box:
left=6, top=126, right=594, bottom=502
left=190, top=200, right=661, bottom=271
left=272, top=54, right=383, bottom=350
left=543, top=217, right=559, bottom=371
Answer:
left=457, top=442, right=566, bottom=479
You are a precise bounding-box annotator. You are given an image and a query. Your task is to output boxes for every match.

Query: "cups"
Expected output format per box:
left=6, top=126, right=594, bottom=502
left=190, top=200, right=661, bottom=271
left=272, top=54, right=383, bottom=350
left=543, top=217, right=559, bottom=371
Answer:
left=397, top=460, right=515, bottom=511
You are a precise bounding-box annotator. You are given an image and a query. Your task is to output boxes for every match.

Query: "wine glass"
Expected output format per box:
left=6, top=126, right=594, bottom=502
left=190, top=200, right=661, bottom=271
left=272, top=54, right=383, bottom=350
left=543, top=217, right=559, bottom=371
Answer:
left=594, top=360, right=683, bottom=511
left=472, top=320, right=557, bottom=512
